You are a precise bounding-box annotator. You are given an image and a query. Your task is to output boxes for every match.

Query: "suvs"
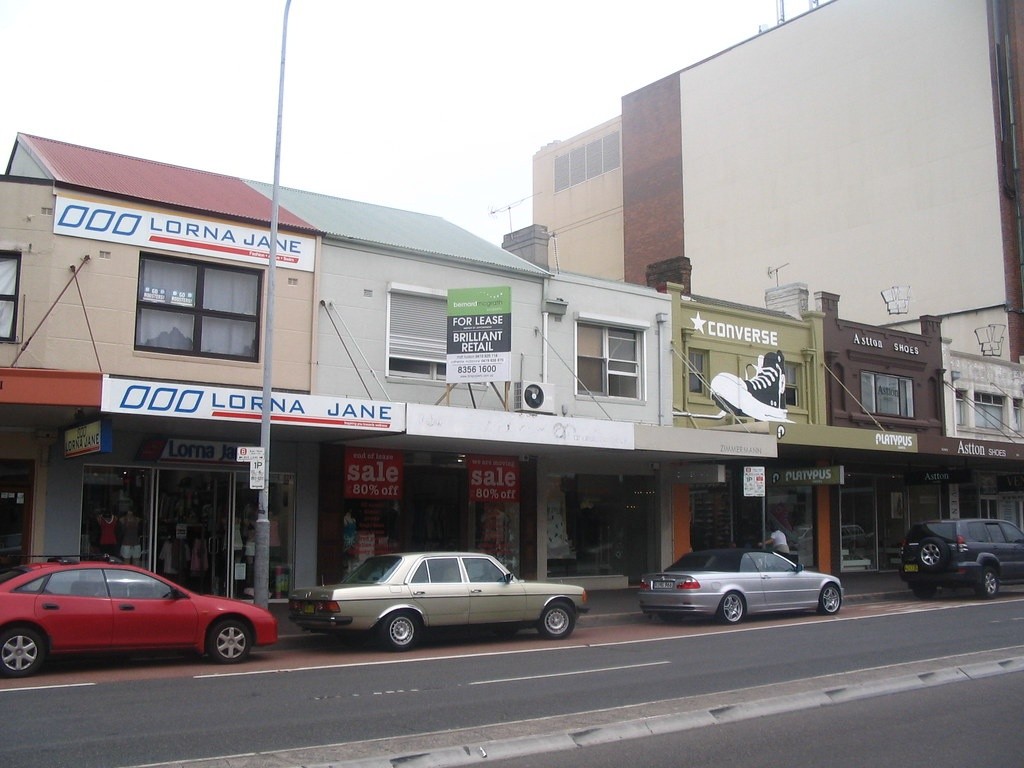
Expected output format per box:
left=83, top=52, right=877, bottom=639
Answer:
left=841, top=525, right=866, bottom=554
left=898, top=518, right=1024, bottom=600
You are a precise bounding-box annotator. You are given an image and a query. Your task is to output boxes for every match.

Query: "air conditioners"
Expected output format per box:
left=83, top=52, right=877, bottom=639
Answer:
left=513, top=380, right=555, bottom=414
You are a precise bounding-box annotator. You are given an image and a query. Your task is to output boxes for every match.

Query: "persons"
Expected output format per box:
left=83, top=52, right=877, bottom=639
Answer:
left=759, top=525, right=789, bottom=554
left=97, top=508, right=119, bottom=546
left=344, top=510, right=356, bottom=547
left=120, top=511, right=143, bottom=565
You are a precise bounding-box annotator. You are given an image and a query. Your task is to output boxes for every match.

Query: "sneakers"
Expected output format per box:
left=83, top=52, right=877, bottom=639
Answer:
left=710, top=350, right=788, bottom=422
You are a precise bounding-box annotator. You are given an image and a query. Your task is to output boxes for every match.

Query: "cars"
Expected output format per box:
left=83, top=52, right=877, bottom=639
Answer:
left=794, top=526, right=813, bottom=552
left=0, top=556, right=278, bottom=680
left=636, top=550, right=844, bottom=626
left=287, top=552, right=590, bottom=653
